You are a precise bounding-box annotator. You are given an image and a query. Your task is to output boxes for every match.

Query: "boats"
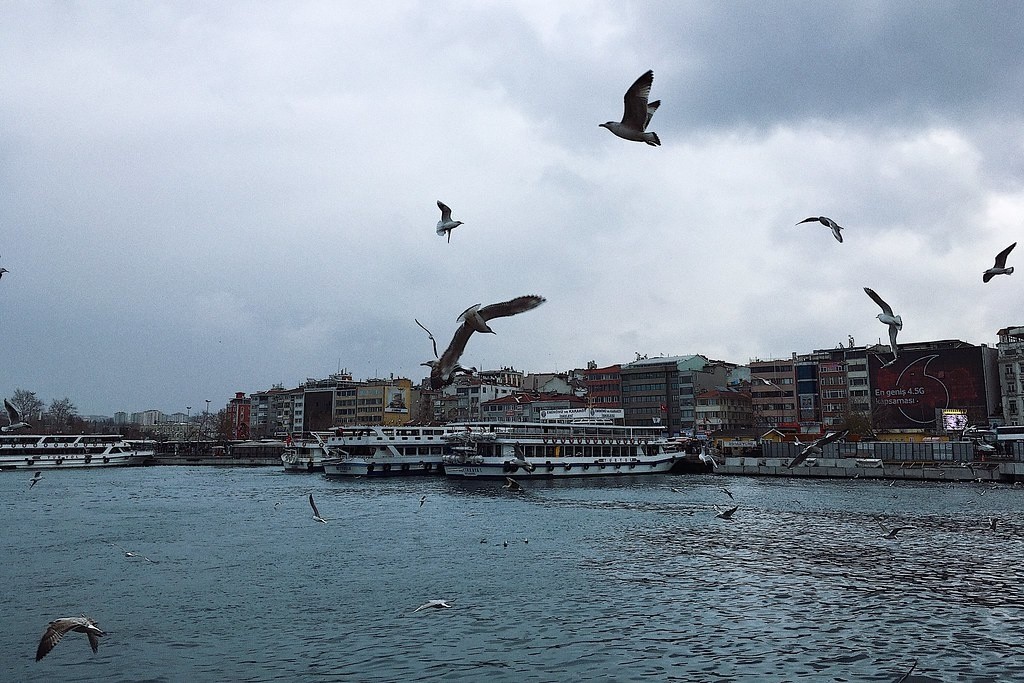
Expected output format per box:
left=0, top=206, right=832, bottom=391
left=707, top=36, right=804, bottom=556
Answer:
left=280, top=431, right=419, bottom=473
left=321, top=425, right=514, bottom=476
left=0, top=435, right=155, bottom=469
left=442, top=421, right=687, bottom=478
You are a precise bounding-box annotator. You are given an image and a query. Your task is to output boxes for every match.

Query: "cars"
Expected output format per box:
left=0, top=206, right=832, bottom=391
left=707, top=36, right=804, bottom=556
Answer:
left=962, top=437, right=997, bottom=456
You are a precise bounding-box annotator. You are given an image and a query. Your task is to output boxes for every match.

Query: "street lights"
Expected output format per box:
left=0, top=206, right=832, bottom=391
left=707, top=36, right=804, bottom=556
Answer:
left=205, top=399, right=211, bottom=441
left=56, top=429, right=63, bottom=435
left=81, top=429, right=85, bottom=434
left=187, top=407, right=191, bottom=432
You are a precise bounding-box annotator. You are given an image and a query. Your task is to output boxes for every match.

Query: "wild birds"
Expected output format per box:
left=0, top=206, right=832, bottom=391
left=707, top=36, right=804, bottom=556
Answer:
left=598, top=68, right=661, bottom=148
left=502, top=539, right=511, bottom=548
left=479, top=537, right=488, bottom=545
left=982, top=242, right=1017, bottom=283
left=0, top=254, right=10, bottom=279
left=795, top=216, right=845, bottom=244
left=501, top=476, right=526, bottom=494
left=832, top=657, right=918, bottom=683
left=687, top=372, right=1024, bottom=573
left=100, top=538, right=160, bottom=566
left=274, top=502, right=282, bottom=507
left=309, top=493, right=343, bottom=524
left=419, top=495, right=427, bottom=507
left=434, top=199, right=464, bottom=244
left=862, top=286, right=904, bottom=359
left=36, top=613, right=108, bottom=663
left=502, top=441, right=535, bottom=474
left=28, top=471, right=46, bottom=490
left=1, top=397, right=33, bottom=432
left=524, top=537, right=530, bottom=544
left=409, top=293, right=549, bottom=391
left=411, top=597, right=461, bottom=614
